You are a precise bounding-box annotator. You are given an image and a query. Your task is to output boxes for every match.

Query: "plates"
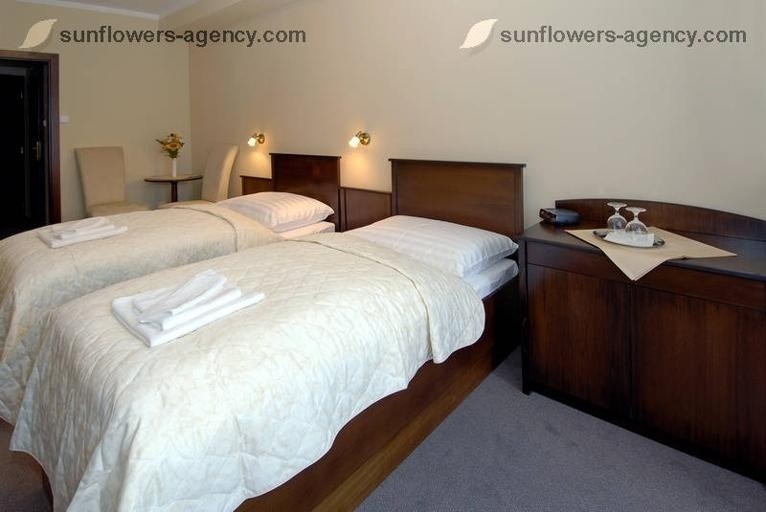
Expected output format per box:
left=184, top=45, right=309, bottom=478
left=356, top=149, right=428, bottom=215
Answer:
left=596, top=230, right=665, bottom=248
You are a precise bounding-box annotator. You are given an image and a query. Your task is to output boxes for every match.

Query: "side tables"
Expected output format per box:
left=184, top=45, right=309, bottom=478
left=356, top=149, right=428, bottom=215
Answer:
left=144, top=176, right=203, bottom=203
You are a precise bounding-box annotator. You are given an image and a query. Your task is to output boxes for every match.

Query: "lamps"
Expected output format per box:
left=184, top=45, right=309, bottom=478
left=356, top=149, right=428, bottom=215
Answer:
left=349, top=131, right=370, bottom=148
left=248, top=134, right=265, bottom=147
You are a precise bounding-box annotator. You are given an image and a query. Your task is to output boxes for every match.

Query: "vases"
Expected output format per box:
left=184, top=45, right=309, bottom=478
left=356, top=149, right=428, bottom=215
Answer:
left=171, top=159, right=176, bottom=177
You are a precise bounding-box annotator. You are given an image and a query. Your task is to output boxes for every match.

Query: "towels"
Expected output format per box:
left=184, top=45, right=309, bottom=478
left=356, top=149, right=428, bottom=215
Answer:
left=37, top=218, right=127, bottom=249
left=112, top=270, right=265, bottom=348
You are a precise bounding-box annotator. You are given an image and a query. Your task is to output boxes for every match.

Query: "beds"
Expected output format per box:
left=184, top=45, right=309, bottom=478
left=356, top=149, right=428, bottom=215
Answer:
left=0, top=159, right=526, bottom=512
left=0, top=153, right=342, bottom=427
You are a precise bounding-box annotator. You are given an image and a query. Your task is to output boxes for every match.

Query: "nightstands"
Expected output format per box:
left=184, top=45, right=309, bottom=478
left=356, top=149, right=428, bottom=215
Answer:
left=519, top=199, right=766, bottom=489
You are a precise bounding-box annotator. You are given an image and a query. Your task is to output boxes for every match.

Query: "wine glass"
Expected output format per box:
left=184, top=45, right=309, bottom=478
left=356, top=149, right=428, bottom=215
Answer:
left=607, top=200, right=628, bottom=234
left=623, top=207, right=649, bottom=243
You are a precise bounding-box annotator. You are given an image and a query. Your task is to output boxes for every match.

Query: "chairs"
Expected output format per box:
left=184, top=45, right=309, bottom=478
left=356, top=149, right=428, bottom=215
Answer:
left=74, top=147, right=148, bottom=217
left=159, top=144, right=239, bottom=209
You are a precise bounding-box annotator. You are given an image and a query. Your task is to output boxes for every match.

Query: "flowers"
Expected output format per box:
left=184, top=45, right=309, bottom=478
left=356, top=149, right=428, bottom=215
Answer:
left=155, top=133, right=185, bottom=158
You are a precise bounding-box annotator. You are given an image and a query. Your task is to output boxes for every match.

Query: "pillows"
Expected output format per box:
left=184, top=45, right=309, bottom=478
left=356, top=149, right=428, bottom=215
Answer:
left=217, top=192, right=334, bottom=233
left=345, top=215, right=519, bottom=279
